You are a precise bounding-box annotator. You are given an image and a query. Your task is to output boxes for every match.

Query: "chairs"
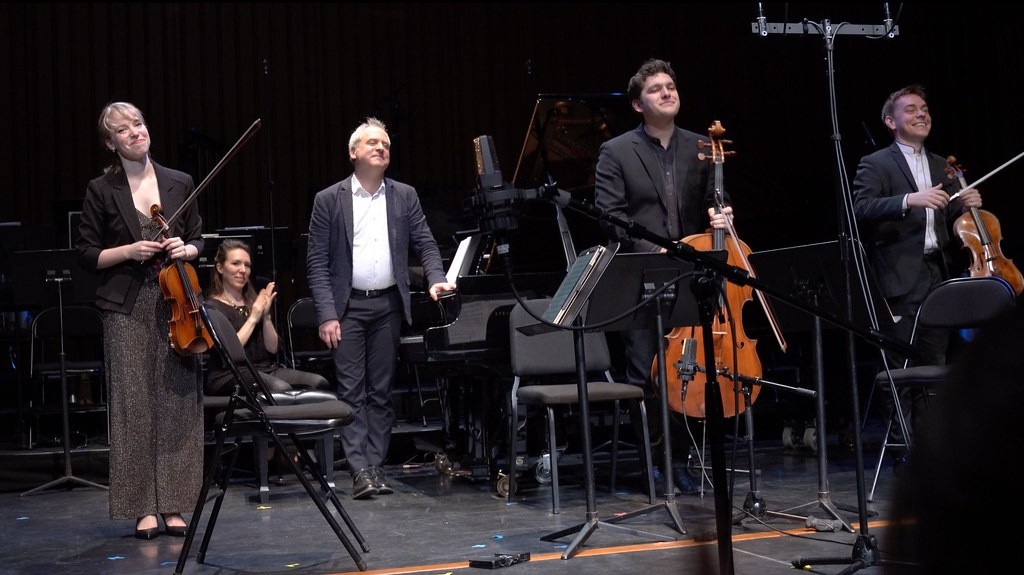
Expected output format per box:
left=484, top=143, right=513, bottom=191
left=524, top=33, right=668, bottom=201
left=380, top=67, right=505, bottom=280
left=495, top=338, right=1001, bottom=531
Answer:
left=864, top=277, right=1013, bottom=507
left=503, top=292, right=660, bottom=514
left=30, top=292, right=460, bottom=504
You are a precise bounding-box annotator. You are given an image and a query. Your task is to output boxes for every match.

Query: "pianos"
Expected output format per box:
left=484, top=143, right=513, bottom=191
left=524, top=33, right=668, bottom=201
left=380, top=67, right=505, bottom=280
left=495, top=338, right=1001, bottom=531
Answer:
left=390, top=227, right=580, bottom=501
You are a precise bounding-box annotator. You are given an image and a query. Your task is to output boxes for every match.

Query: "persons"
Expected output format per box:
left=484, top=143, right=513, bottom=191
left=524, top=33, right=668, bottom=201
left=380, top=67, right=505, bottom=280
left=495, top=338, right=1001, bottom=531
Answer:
left=593, top=58, right=734, bottom=496
left=305, top=117, right=457, bottom=499
left=198, top=239, right=331, bottom=489
left=852, top=85, right=982, bottom=493
left=76, top=101, right=204, bottom=540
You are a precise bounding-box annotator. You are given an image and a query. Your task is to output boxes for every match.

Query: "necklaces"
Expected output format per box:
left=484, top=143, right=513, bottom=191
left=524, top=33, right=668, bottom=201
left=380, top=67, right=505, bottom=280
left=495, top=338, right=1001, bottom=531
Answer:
left=223, top=293, right=251, bottom=318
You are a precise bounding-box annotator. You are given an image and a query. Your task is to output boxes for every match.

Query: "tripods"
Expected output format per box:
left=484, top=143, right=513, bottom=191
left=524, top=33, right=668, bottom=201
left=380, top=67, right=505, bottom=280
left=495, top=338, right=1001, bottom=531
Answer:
left=515, top=0, right=919, bottom=575
left=0, top=249, right=110, bottom=498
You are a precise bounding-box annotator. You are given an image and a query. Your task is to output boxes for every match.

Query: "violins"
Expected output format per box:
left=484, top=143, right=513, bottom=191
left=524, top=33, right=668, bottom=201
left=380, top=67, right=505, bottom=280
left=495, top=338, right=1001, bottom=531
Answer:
left=151, top=203, right=215, bottom=356
left=946, top=155, right=1024, bottom=297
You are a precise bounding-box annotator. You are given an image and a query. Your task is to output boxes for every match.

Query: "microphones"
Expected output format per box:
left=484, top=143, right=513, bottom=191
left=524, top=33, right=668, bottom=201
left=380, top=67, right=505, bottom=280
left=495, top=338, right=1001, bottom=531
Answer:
left=472, top=133, right=514, bottom=282
left=680, top=338, right=698, bottom=401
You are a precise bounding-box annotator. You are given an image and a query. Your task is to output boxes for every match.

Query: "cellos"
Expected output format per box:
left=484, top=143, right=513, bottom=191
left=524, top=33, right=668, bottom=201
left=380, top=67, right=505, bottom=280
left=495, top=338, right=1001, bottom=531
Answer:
left=651, top=119, right=764, bottom=498
